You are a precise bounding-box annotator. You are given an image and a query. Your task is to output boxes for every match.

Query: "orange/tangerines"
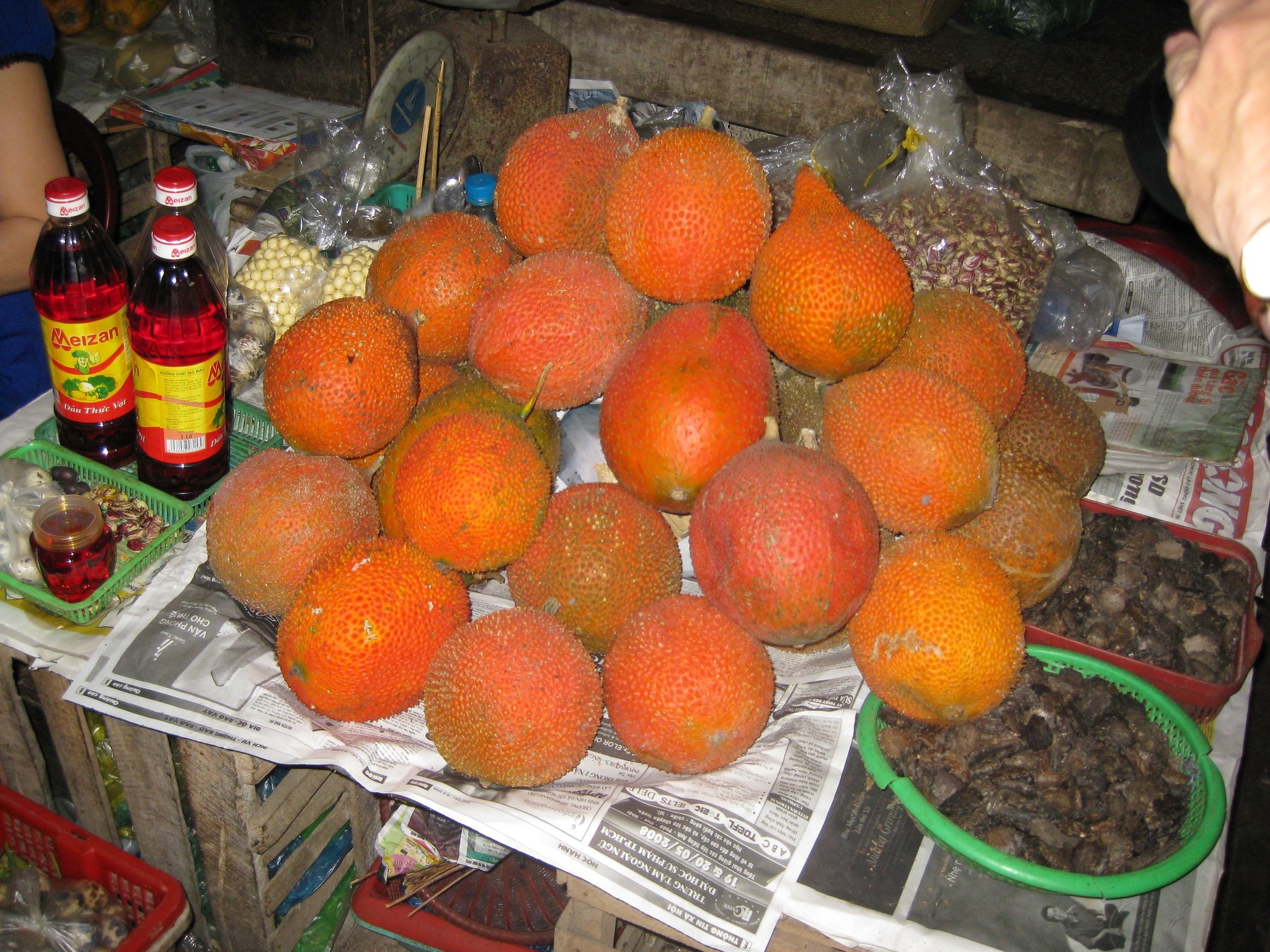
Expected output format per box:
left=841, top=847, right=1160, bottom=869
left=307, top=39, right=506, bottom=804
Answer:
left=205, top=95, right=1106, bottom=784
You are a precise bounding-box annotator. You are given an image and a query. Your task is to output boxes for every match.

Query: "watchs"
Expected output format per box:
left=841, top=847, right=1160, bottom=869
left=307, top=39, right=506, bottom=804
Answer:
left=1241, top=218, right=1270, bottom=330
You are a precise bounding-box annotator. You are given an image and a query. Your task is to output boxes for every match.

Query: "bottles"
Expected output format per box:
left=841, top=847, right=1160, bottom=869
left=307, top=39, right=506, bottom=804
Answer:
left=27, top=177, right=139, bottom=469
left=28, top=494, right=117, bottom=603
left=127, top=215, right=232, bottom=501
left=462, top=172, right=498, bottom=224
left=131, top=167, right=229, bottom=300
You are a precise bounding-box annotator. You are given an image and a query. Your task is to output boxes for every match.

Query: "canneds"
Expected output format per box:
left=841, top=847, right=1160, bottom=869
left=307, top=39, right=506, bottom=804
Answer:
left=27, top=496, right=116, bottom=604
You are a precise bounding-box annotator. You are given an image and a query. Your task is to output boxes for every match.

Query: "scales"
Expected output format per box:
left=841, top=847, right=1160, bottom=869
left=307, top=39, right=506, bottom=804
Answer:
left=357, top=0, right=572, bottom=212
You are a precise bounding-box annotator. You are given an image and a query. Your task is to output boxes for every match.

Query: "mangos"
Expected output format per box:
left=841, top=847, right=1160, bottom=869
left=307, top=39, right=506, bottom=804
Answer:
left=103, top=37, right=196, bottom=90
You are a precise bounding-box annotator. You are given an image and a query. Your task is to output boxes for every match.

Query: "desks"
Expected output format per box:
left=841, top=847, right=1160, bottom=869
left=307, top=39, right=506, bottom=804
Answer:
left=0, top=222, right=1270, bottom=952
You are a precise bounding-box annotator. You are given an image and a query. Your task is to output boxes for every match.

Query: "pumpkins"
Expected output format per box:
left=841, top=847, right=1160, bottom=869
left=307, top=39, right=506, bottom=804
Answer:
left=44, top=0, right=173, bottom=35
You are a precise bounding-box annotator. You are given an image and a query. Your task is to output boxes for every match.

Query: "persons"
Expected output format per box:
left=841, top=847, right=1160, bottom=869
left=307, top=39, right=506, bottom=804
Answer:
left=1165, top=0, right=1270, bottom=338
left=0, top=0, right=69, bottom=429
